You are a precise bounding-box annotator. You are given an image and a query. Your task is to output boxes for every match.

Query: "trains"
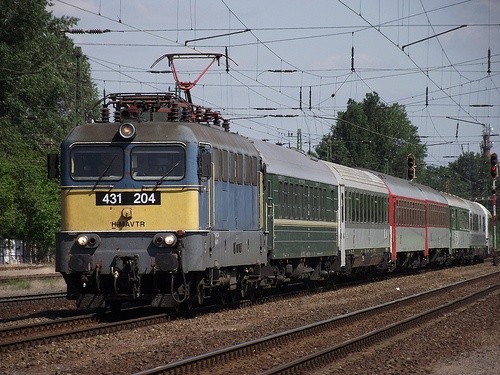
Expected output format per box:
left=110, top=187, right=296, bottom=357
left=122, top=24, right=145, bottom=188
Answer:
left=47, top=92, right=492, bottom=315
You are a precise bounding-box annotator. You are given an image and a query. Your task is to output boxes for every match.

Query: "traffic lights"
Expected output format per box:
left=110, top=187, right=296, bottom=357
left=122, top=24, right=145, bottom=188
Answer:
left=491, top=153, right=498, bottom=179
left=407, top=154, right=417, bottom=182
